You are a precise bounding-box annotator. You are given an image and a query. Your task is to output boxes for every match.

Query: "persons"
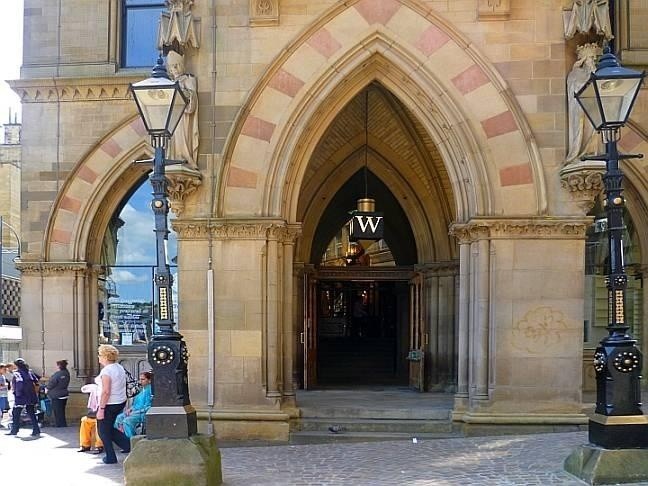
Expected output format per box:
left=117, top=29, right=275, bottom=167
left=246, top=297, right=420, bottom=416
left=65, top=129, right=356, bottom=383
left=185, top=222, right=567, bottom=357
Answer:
left=77, top=410, right=104, bottom=454
left=566, top=42, right=604, bottom=163
left=114, top=372, right=152, bottom=453
left=164, top=51, right=197, bottom=168
left=0, top=359, right=41, bottom=436
left=46, top=361, right=70, bottom=427
left=95, top=343, right=130, bottom=464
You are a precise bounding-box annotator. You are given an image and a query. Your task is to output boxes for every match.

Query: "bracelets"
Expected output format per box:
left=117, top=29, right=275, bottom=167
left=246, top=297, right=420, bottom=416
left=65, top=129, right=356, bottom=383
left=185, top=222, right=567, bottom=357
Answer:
left=98, top=407, right=105, bottom=409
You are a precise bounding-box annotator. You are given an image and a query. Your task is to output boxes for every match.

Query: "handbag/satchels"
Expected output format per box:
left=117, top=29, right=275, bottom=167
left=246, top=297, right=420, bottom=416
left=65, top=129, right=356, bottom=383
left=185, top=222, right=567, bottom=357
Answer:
left=125, top=371, right=141, bottom=398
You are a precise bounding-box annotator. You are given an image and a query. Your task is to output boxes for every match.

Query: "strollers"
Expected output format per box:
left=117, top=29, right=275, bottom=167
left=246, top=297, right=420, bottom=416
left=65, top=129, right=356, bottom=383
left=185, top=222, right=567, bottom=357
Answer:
left=8, top=384, right=52, bottom=431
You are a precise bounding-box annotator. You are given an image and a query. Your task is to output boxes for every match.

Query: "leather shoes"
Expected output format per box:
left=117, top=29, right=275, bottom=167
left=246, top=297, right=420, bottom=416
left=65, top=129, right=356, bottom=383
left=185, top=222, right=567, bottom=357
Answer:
left=77, top=446, right=90, bottom=452
left=4, top=431, right=18, bottom=435
left=119, top=449, right=130, bottom=453
left=93, top=447, right=102, bottom=454
left=31, top=429, right=40, bottom=436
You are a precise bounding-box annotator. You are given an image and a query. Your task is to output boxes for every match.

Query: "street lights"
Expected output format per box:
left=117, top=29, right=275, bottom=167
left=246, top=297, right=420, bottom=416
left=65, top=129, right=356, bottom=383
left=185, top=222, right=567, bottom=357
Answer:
left=573, top=40, right=647, bottom=449
left=129, top=50, right=196, bottom=440
